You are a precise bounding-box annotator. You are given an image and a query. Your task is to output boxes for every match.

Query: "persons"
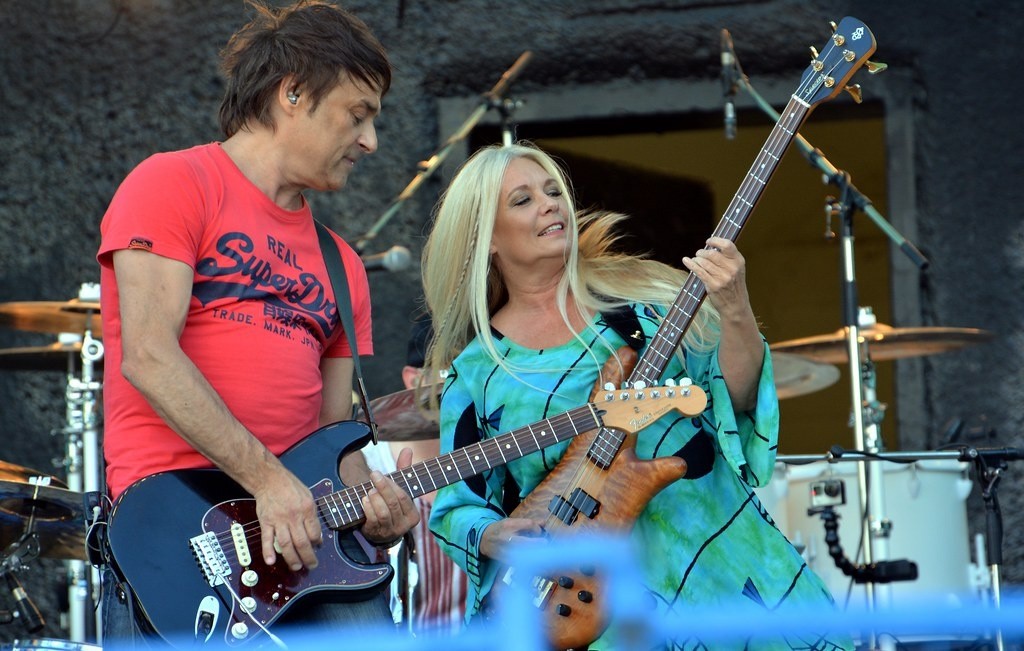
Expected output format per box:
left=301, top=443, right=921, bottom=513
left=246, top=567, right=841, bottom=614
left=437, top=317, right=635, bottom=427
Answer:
left=96, top=0, right=420, bottom=645
left=423, top=143, right=857, bottom=651
left=359, top=310, right=469, bottom=637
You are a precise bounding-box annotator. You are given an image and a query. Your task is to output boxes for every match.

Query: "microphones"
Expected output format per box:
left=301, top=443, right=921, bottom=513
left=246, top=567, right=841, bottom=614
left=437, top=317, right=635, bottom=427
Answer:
left=720, top=33, right=737, bottom=139
left=360, top=245, right=411, bottom=272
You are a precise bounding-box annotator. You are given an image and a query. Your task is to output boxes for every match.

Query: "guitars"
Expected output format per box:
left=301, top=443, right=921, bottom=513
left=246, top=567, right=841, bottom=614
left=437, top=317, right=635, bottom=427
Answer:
left=483, top=18, right=880, bottom=646
left=102, top=376, right=708, bottom=651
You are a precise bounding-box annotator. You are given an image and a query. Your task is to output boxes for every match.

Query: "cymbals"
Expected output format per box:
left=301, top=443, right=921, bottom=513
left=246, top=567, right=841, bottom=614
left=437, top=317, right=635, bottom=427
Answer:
left=356, top=383, right=443, bottom=443
left=773, top=324, right=994, bottom=361
left=0, top=343, right=104, bottom=373
left=766, top=337, right=842, bottom=400
left=0, top=479, right=89, bottom=560
left=0, top=460, right=69, bottom=490
left=0, top=296, right=103, bottom=340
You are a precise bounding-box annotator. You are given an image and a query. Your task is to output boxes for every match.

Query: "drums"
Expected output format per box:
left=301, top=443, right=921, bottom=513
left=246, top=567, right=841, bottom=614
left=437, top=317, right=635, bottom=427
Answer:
left=786, top=454, right=986, bottom=651
left=748, top=461, right=788, bottom=540
left=0, top=638, right=103, bottom=651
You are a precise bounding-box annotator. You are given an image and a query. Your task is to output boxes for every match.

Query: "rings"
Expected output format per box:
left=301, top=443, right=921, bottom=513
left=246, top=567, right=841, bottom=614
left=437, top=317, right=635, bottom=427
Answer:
left=507, top=535, right=515, bottom=544
